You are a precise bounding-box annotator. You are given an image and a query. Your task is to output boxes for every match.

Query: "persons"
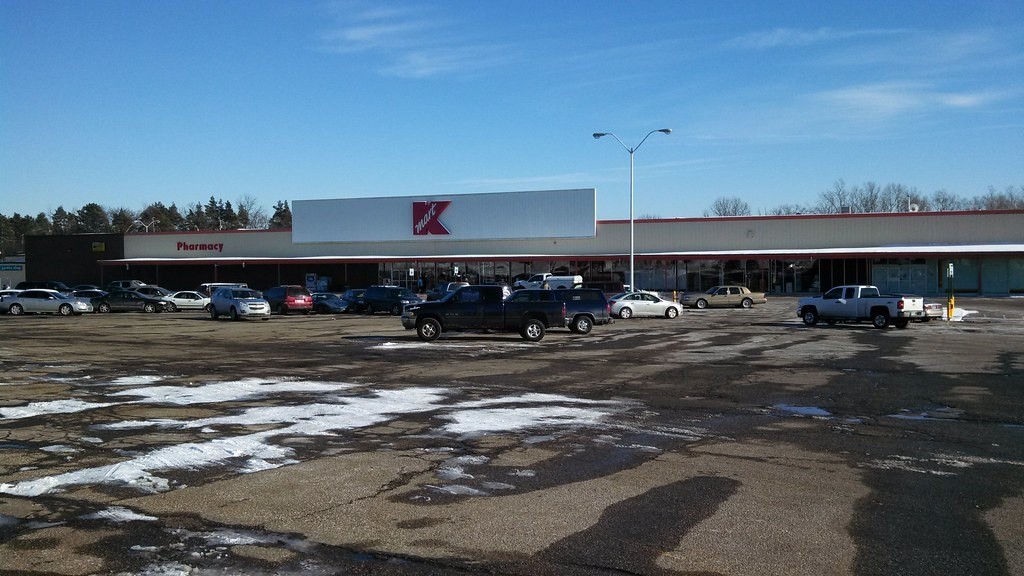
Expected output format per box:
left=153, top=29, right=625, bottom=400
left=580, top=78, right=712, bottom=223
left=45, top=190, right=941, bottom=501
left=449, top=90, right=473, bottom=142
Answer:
left=541, top=279, right=551, bottom=290
left=462, top=272, right=468, bottom=282
left=417, top=273, right=427, bottom=294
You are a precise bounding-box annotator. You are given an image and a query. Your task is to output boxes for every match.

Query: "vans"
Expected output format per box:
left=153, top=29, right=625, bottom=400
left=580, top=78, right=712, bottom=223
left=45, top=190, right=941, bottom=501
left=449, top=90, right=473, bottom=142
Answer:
left=503, top=288, right=614, bottom=334
left=569, top=281, right=624, bottom=299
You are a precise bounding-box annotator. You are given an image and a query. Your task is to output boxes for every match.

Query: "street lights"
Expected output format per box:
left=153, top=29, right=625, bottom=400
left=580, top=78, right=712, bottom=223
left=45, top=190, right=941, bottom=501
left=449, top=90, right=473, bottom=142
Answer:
left=134, top=220, right=160, bottom=232
left=593, top=128, right=673, bottom=292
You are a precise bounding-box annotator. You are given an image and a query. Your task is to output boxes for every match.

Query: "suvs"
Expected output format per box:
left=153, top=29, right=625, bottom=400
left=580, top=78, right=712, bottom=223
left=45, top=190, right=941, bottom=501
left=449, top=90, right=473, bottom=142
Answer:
left=363, top=285, right=425, bottom=316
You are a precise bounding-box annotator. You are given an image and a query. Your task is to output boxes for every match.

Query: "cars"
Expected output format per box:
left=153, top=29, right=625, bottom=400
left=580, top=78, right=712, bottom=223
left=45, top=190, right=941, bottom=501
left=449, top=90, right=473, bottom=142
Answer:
left=263, top=285, right=313, bottom=315
left=623, top=285, right=658, bottom=298
left=211, top=287, right=271, bottom=321
left=607, top=292, right=683, bottom=319
left=426, top=281, right=479, bottom=301
left=0, top=291, right=94, bottom=316
left=0, top=279, right=173, bottom=314
left=678, top=285, right=767, bottom=309
left=921, top=302, right=943, bottom=322
left=161, top=291, right=211, bottom=312
left=340, top=289, right=367, bottom=314
left=311, top=293, right=351, bottom=314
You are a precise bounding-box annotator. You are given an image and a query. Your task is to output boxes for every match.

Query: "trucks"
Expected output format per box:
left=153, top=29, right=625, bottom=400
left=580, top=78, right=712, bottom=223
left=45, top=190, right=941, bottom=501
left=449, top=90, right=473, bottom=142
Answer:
left=512, top=273, right=583, bottom=290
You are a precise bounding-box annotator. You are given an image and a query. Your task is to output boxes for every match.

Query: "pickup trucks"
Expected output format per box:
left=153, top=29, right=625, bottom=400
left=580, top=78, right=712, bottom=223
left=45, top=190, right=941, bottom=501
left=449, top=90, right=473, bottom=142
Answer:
left=401, top=285, right=570, bottom=342
left=797, top=285, right=924, bottom=330
